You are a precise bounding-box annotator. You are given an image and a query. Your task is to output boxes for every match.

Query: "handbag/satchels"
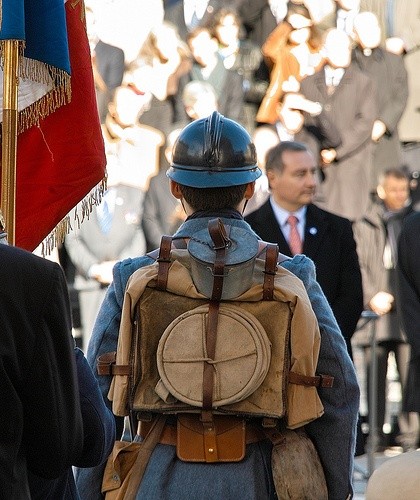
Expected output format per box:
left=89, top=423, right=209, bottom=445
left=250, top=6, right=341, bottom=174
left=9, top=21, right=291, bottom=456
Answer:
left=102, top=439, right=156, bottom=500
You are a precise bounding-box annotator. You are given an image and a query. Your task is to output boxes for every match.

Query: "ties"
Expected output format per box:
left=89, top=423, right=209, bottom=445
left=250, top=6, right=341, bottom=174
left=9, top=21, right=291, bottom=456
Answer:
left=288, top=216, right=302, bottom=257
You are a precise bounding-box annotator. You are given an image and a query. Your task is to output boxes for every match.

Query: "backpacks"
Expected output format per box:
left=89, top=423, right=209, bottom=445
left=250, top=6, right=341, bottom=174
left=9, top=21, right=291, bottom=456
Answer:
left=93, top=218, right=339, bottom=432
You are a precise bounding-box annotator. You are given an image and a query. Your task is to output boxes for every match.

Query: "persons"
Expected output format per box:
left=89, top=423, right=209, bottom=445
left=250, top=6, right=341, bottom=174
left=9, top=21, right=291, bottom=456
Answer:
left=350, top=168, right=409, bottom=452
left=66, top=152, right=144, bottom=356
left=30, top=0, right=420, bottom=266
left=76, top=111, right=360, bottom=500
left=365, top=454, right=419, bottom=499
left=244, top=143, right=365, bottom=362
left=396, top=168, right=420, bottom=453
left=0, top=244, right=115, bottom=500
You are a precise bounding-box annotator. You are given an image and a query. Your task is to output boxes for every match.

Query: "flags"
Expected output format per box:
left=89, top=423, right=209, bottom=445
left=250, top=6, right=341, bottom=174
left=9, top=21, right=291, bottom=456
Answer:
left=0, top=0, right=107, bottom=254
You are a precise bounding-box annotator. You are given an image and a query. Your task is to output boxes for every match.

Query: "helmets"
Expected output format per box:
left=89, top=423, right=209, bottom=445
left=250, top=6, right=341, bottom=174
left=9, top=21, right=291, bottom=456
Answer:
left=165, top=110, right=263, bottom=190
